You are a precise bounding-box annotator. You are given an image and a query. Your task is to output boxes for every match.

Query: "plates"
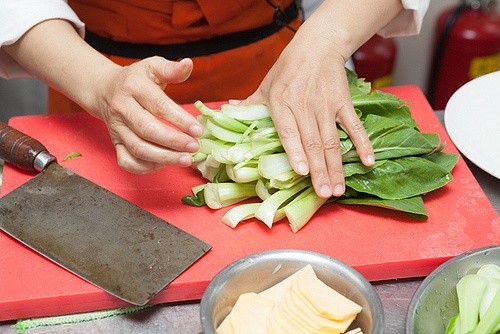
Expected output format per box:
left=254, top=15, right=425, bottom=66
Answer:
left=443, top=67, right=500, bottom=180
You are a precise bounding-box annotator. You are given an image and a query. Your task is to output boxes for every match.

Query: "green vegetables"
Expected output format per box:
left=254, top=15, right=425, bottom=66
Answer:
left=181, top=65, right=460, bottom=233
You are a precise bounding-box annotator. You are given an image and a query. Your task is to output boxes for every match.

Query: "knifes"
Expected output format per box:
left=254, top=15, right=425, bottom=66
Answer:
left=0, top=117, right=213, bottom=307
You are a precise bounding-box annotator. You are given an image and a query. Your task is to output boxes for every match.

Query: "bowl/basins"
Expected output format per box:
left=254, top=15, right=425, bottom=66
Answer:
left=405, top=246, right=500, bottom=334
left=198, top=248, right=384, bottom=334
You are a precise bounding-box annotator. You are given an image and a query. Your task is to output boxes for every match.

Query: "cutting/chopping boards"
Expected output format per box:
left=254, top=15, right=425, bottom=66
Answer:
left=0, top=83, right=500, bottom=324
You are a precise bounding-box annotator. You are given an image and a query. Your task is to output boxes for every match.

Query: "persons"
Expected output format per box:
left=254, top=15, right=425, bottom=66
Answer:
left=0, top=0, right=433, bottom=199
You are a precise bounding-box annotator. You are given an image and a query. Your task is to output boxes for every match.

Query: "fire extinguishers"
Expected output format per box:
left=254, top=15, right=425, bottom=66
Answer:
left=349, top=32, right=395, bottom=90
left=426, top=0, right=499, bottom=112
